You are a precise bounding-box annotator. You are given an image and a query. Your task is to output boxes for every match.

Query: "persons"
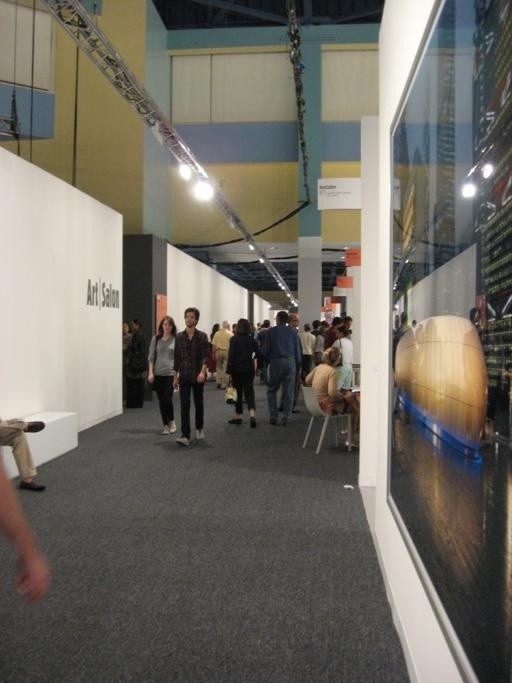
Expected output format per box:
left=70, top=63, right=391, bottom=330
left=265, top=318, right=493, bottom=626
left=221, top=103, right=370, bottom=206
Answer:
left=210, top=310, right=360, bottom=449
left=123, top=321, right=133, bottom=355
left=147, top=315, right=179, bottom=434
left=461, top=293, right=501, bottom=439
left=125, top=317, right=150, bottom=408
left=392, top=309, right=442, bottom=424
left=0, top=457, right=50, bottom=602
left=173, top=306, right=212, bottom=446
left=1, top=417, right=47, bottom=490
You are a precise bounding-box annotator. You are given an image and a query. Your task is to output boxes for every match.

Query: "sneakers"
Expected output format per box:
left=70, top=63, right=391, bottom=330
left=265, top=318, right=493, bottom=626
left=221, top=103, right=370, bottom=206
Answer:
left=161, top=424, right=169, bottom=435
left=195, top=427, right=206, bottom=440
left=175, top=435, right=192, bottom=447
left=24, top=420, right=45, bottom=433
left=170, top=419, right=177, bottom=434
left=20, top=479, right=47, bottom=492
left=228, top=417, right=242, bottom=425
left=249, top=416, right=258, bottom=429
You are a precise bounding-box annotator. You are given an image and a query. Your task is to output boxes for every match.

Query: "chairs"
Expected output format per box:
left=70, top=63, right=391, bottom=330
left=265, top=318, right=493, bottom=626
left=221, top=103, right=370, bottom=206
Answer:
left=297, top=381, right=354, bottom=455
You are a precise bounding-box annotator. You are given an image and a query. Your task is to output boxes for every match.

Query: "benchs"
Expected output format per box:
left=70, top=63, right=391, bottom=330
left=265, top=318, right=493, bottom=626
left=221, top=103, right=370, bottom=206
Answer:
left=0, top=409, right=80, bottom=487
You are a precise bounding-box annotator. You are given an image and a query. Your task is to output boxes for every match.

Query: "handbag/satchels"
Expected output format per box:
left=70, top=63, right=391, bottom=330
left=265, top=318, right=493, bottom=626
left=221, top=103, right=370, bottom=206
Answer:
left=223, top=387, right=238, bottom=406
left=128, top=347, right=147, bottom=376
